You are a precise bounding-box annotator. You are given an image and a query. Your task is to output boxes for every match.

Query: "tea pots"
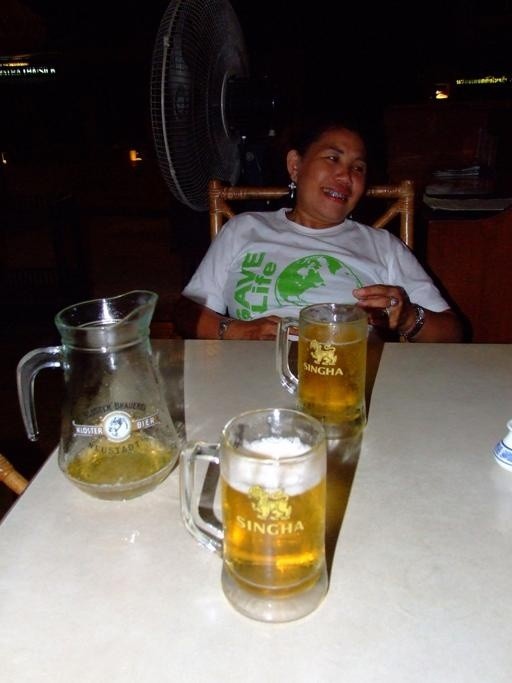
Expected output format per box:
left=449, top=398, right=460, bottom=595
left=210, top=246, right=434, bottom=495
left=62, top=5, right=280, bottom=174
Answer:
left=16, top=288, right=178, bottom=499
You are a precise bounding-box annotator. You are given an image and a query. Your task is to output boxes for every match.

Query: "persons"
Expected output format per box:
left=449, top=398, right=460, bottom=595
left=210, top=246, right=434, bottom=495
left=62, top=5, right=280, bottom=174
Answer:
left=170, top=114, right=466, bottom=343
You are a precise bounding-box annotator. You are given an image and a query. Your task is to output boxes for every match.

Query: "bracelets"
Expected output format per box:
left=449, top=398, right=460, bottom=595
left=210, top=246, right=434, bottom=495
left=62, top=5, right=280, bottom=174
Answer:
left=405, top=304, right=425, bottom=340
left=217, top=318, right=234, bottom=339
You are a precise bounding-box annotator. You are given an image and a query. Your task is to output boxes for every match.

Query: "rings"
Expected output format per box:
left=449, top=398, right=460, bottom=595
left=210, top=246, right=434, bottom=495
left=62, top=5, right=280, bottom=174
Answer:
left=384, top=310, right=390, bottom=320
left=388, top=295, right=399, bottom=308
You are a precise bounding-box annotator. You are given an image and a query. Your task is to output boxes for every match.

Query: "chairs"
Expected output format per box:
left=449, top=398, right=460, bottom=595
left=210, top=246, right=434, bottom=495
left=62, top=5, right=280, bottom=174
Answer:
left=208, top=177, right=416, bottom=255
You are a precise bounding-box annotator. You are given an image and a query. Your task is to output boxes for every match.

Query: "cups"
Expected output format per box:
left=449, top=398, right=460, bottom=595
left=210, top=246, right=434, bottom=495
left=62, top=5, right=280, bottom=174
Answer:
left=274, top=300, right=367, bottom=439
left=176, top=401, right=335, bottom=620
